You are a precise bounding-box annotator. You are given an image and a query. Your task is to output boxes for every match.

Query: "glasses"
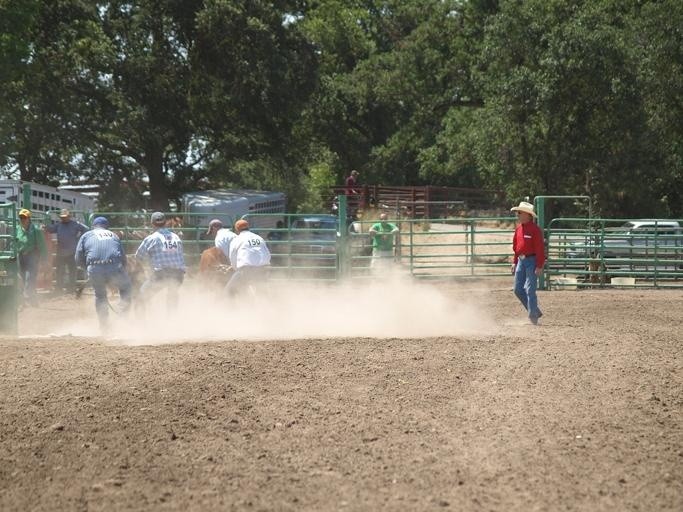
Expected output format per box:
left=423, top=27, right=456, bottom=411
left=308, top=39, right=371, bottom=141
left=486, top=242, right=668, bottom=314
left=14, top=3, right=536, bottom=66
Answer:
left=514, top=211, right=528, bottom=215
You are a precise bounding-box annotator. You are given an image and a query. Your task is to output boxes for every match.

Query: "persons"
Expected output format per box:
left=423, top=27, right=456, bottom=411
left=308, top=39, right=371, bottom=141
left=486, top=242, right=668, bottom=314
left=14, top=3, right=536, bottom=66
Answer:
left=344, top=170, right=361, bottom=221
left=510, top=202, right=546, bottom=325
left=398, top=206, right=416, bottom=224
left=226, top=220, right=272, bottom=297
left=206, top=219, right=239, bottom=259
left=74, top=217, right=133, bottom=337
left=332, top=196, right=354, bottom=232
left=369, top=214, right=400, bottom=275
left=44, top=209, right=91, bottom=295
left=10, top=209, right=50, bottom=297
left=574, top=257, right=613, bottom=290
left=136, top=212, right=186, bottom=299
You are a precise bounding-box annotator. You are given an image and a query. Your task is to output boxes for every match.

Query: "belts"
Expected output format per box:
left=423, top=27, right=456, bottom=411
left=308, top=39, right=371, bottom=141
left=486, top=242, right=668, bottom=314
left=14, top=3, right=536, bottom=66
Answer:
left=517, top=254, right=536, bottom=259
left=88, top=259, right=122, bottom=262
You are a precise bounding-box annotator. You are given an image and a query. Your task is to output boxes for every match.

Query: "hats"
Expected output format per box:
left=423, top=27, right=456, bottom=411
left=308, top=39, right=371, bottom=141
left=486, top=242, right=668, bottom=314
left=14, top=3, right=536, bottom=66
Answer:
left=511, top=200, right=538, bottom=219
left=92, top=217, right=108, bottom=225
left=18, top=209, right=32, bottom=219
left=206, top=219, right=222, bottom=235
left=58, top=208, right=73, bottom=219
left=234, top=220, right=251, bottom=232
left=149, top=212, right=166, bottom=226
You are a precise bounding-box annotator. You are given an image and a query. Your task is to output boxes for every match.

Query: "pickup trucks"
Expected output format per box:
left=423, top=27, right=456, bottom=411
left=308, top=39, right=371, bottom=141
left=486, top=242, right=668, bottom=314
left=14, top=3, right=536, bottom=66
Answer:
left=565, top=220, right=683, bottom=271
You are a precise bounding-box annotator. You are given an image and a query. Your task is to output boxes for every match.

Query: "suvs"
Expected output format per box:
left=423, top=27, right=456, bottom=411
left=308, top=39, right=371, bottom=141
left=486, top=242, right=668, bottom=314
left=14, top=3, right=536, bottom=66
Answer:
left=276, top=216, right=360, bottom=278
